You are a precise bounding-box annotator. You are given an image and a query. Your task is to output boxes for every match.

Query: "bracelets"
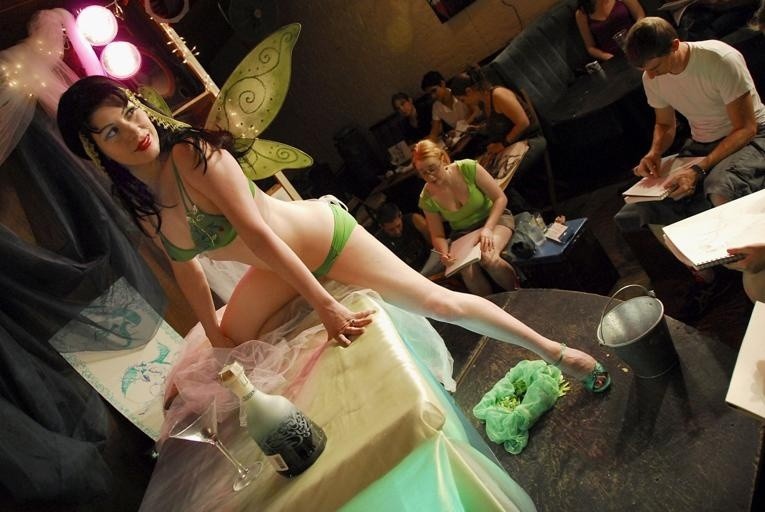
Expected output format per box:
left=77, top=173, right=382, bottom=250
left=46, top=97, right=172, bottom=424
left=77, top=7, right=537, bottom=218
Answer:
left=690, top=164, right=706, bottom=183
left=498, top=138, right=510, bottom=148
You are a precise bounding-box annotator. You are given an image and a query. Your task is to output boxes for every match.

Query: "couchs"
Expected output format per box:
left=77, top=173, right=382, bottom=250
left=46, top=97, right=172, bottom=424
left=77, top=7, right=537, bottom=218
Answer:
left=365, top=0, right=702, bottom=163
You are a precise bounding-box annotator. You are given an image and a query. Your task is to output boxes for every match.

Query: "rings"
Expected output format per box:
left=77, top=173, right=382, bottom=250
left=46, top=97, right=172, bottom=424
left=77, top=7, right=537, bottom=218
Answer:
left=352, top=319, right=356, bottom=327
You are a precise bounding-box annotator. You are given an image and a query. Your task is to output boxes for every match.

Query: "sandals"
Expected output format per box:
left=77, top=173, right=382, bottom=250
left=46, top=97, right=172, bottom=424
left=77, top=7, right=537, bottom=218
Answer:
left=552, top=340, right=613, bottom=394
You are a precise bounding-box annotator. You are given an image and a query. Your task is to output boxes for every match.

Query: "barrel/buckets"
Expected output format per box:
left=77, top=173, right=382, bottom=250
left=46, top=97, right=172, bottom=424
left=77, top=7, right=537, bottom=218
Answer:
left=597, top=285, right=676, bottom=380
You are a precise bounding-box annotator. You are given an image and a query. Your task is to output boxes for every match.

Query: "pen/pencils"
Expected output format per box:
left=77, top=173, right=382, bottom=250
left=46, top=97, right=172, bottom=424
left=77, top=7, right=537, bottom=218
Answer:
left=430, top=250, right=457, bottom=260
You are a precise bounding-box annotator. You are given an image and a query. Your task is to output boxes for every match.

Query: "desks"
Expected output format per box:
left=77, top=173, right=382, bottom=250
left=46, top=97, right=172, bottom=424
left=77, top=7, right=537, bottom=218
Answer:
left=142, top=277, right=543, bottom=512
left=513, top=216, right=620, bottom=297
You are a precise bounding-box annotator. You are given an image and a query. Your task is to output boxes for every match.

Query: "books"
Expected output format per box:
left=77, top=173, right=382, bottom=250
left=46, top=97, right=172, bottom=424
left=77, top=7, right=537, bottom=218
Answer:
left=622, top=155, right=706, bottom=205
left=444, top=227, right=482, bottom=278
left=658, top=1, right=698, bottom=27
left=660, top=187, right=765, bottom=273
left=475, top=141, right=529, bottom=193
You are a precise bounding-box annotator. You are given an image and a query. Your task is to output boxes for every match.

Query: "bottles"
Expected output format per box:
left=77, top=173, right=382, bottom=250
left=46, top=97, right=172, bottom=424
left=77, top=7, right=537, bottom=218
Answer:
left=218, top=363, right=327, bottom=480
left=441, top=132, right=456, bottom=151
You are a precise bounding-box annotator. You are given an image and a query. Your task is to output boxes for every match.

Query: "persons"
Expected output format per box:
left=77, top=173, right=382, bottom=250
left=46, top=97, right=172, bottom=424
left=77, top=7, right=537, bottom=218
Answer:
left=53, top=76, right=612, bottom=396
left=418, top=69, right=482, bottom=142
left=370, top=201, right=436, bottom=273
left=615, top=17, right=765, bottom=318
left=716, top=245, right=764, bottom=305
left=391, top=92, right=434, bottom=140
left=449, top=61, right=550, bottom=213
left=573, top=1, right=647, bottom=71
left=407, top=136, right=523, bottom=295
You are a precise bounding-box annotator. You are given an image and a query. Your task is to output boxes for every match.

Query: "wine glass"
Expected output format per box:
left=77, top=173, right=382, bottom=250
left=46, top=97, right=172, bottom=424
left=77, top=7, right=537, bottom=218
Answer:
left=168, top=395, right=263, bottom=492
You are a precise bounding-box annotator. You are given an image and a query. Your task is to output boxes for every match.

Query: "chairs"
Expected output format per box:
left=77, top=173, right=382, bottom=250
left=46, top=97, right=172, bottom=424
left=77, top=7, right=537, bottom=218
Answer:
left=332, top=86, right=556, bottom=232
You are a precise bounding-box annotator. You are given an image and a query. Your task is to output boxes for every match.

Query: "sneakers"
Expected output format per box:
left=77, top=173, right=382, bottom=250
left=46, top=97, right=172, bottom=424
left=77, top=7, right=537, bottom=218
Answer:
left=676, top=275, right=735, bottom=322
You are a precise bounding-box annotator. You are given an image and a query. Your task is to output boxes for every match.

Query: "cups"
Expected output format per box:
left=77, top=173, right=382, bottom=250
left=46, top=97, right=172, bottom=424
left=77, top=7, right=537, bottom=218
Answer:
left=613, top=29, right=628, bottom=51
left=521, top=215, right=548, bottom=247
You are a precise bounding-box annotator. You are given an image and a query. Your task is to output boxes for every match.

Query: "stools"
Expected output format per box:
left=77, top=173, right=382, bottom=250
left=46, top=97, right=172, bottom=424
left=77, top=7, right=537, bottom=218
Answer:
left=612, top=204, right=693, bottom=285
